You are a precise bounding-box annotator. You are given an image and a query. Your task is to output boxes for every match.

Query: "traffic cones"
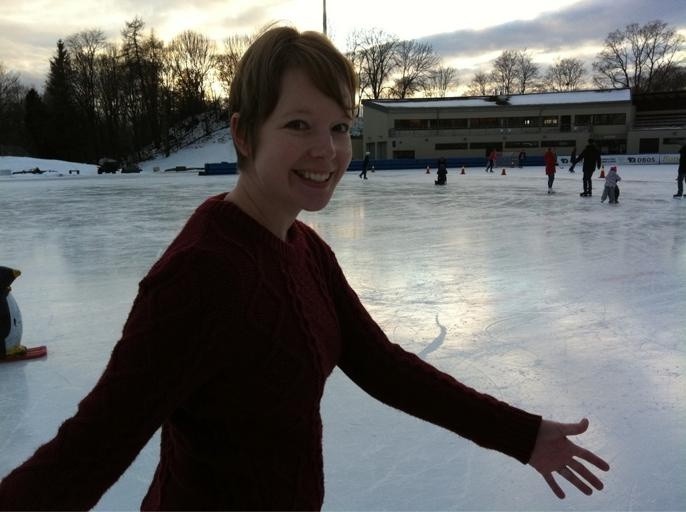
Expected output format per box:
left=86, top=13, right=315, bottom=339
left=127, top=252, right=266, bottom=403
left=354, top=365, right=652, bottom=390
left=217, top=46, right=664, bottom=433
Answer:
left=501, top=167, right=508, bottom=175
left=460, top=165, right=466, bottom=175
left=598, top=164, right=606, bottom=178
left=425, top=166, right=431, bottom=175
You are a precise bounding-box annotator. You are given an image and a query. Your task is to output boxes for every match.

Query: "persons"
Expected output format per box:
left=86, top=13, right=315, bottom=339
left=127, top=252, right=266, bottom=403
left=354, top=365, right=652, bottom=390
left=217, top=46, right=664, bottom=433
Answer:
left=518, top=149, right=527, bottom=168
left=601, top=167, right=621, bottom=204
left=486, top=148, right=496, bottom=172
left=436, top=162, right=447, bottom=185
left=1, top=25, right=609, bottom=511
left=570, top=147, right=576, bottom=162
left=676, top=139, right=686, bottom=196
left=544, top=147, right=556, bottom=194
left=360, top=151, right=370, bottom=180
left=569, top=138, right=601, bottom=198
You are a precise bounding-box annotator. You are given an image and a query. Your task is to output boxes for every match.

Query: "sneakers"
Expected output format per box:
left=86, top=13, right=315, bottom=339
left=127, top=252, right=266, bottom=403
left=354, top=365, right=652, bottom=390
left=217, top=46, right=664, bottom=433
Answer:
left=671, top=192, right=683, bottom=198
left=545, top=187, right=592, bottom=198
